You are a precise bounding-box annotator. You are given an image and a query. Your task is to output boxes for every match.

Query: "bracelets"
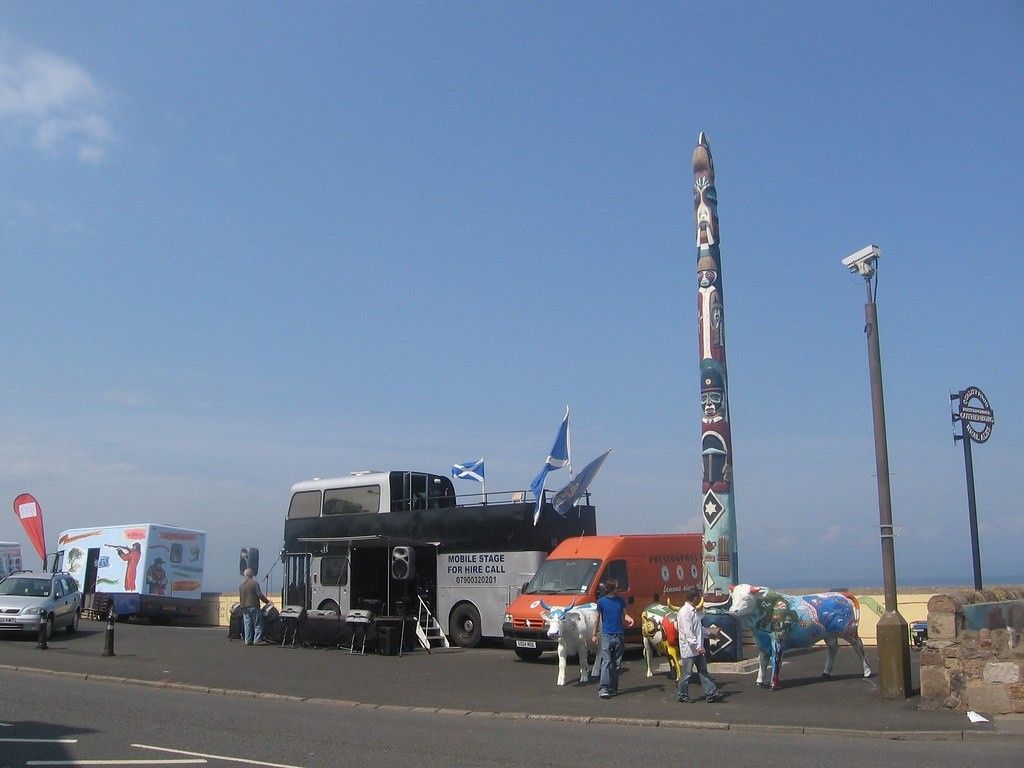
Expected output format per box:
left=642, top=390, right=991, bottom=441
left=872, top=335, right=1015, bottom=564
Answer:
left=593, top=634, right=596, bottom=636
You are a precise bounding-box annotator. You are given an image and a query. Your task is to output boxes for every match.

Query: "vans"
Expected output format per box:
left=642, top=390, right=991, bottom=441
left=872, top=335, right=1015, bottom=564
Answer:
left=500, top=531, right=706, bottom=661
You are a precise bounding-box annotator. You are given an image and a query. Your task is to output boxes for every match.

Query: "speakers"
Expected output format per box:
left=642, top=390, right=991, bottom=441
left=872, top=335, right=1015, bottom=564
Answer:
left=239, top=548, right=259, bottom=577
left=378, top=627, right=399, bottom=655
left=391, top=546, right=416, bottom=580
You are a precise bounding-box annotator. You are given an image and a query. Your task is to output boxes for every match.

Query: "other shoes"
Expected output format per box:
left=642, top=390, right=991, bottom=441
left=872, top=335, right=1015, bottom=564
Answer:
left=706, top=690, right=727, bottom=703
left=679, top=696, right=695, bottom=704
left=245, top=642, right=251, bottom=645
left=252, top=640, right=267, bottom=645
left=598, top=689, right=609, bottom=699
left=610, top=690, right=618, bottom=696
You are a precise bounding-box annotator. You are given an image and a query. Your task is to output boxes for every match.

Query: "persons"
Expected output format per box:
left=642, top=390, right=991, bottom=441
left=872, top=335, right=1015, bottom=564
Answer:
left=592, top=579, right=635, bottom=698
left=239, top=569, right=273, bottom=646
left=676, top=587, right=725, bottom=703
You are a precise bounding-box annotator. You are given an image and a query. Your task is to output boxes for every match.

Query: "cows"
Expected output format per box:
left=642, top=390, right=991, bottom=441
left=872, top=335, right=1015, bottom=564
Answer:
left=727, top=583, right=872, bottom=693
left=641, top=597, right=705, bottom=682
left=539, top=598, right=604, bottom=686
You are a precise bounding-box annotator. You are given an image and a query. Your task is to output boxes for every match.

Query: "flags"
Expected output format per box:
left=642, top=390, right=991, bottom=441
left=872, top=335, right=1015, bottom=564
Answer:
left=530, top=413, right=611, bottom=526
left=451, top=460, right=484, bottom=480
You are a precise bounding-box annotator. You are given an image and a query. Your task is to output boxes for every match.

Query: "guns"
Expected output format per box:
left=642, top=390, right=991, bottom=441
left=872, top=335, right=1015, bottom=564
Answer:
left=104, top=544, right=132, bottom=552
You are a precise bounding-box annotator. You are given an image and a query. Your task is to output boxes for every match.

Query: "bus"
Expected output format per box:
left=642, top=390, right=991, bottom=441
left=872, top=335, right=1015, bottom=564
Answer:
left=278, top=467, right=598, bottom=650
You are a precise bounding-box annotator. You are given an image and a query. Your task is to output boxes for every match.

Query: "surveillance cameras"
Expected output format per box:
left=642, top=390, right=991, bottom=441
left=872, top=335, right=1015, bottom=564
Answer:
left=841, top=245, right=880, bottom=273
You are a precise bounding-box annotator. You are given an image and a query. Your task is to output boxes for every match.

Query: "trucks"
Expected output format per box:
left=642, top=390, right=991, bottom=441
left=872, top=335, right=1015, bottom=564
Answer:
left=0, top=541, right=24, bottom=581
left=57, top=521, right=206, bottom=625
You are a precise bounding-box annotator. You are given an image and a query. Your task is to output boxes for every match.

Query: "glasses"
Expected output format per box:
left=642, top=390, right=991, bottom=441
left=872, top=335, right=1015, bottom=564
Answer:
left=615, top=584, right=621, bottom=590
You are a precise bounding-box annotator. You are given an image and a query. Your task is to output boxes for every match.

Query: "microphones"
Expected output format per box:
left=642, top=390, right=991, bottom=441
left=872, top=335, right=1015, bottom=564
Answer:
left=282, top=540, right=286, bottom=563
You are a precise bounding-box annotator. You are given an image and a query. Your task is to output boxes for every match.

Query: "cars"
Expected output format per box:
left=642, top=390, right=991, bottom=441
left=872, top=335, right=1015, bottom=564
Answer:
left=0, top=567, right=82, bottom=641
